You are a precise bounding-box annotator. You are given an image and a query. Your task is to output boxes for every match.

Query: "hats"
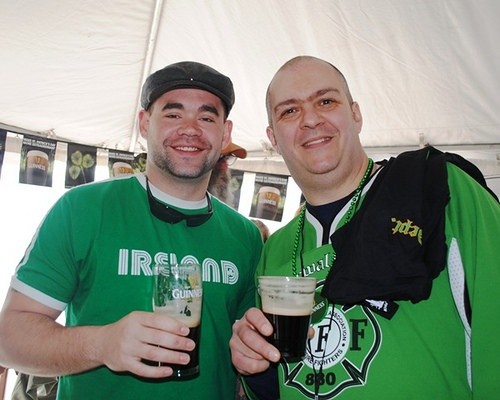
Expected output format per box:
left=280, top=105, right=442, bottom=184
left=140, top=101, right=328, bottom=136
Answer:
left=140, top=61, right=234, bottom=112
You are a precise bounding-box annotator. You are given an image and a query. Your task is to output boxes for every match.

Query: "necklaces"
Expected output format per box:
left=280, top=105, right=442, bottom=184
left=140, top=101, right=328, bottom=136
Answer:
left=291, top=158, right=373, bottom=276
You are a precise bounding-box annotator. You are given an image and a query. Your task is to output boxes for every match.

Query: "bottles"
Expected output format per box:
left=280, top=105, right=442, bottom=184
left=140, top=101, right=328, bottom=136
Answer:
left=152, top=263, right=202, bottom=376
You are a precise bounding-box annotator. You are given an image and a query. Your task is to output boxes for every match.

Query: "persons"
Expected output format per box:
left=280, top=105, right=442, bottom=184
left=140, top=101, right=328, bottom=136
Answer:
left=208, top=140, right=247, bottom=210
left=229, top=56, right=500, bottom=400
left=251, top=219, right=269, bottom=243
left=0, top=60, right=265, bottom=400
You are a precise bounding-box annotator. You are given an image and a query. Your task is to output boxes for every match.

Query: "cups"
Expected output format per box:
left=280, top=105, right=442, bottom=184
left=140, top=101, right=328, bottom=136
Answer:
left=257, top=276, right=317, bottom=362
left=25, top=150, right=49, bottom=186
left=112, top=162, right=133, bottom=179
left=255, top=186, right=281, bottom=221
